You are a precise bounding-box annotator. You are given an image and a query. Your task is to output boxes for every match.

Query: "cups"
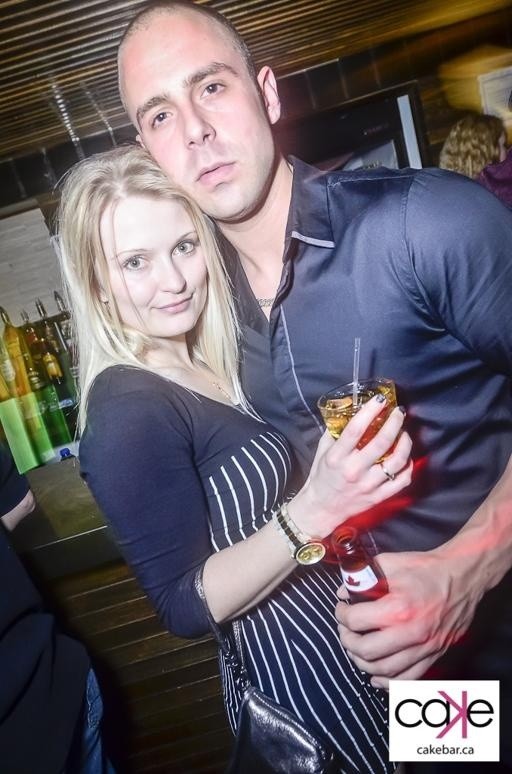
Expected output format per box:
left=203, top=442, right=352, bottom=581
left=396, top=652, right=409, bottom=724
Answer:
left=315, top=375, right=409, bottom=465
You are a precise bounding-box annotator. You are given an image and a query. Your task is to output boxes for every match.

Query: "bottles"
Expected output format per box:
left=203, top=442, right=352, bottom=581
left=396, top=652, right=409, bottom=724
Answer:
left=0, top=290, right=81, bottom=403
left=327, top=531, right=446, bottom=680
left=57, top=446, right=74, bottom=461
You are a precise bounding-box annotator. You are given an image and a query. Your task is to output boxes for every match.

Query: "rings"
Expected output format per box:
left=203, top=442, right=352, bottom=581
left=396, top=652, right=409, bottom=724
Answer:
left=378, top=462, right=395, bottom=481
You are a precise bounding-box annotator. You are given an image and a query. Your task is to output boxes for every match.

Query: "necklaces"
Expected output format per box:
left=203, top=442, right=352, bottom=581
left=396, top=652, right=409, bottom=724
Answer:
left=255, top=297, right=274, bottom=307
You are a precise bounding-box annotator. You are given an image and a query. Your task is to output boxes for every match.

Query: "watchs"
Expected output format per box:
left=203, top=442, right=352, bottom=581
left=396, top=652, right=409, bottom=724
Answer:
left=270, top=504, right=329, bottom=567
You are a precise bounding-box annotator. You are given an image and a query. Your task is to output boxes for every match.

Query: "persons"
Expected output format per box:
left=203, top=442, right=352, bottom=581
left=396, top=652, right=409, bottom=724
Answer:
left=115, top=2, right=511, bottom=771
left=1, top=424, right=124, bottom=773
left=437, top=109, right=508, bottom=180
left=46, top=143, right=418, bottom=772
